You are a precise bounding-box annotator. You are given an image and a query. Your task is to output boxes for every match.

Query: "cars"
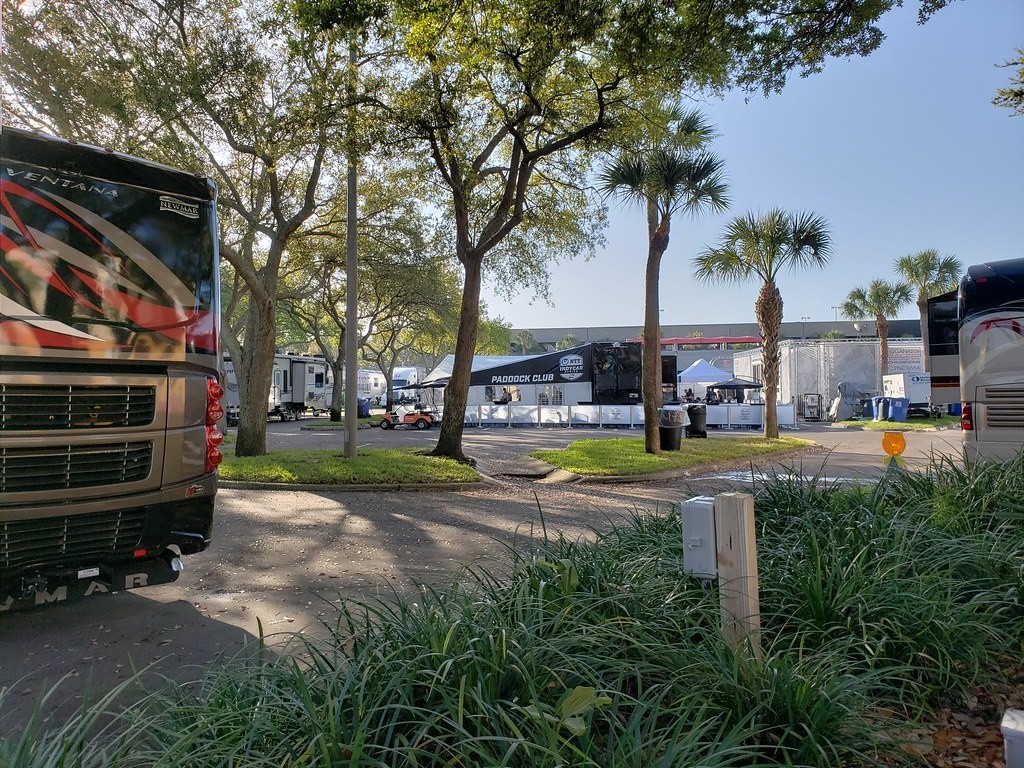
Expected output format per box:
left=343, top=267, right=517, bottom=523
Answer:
left=378, top=390, right=411, bottom=406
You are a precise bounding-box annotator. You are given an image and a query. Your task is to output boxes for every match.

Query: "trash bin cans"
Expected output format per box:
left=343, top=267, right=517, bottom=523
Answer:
left=685, top=405, right=707, bottom=439
left=357, top=399, right=371, bottom=420
left=861, top=395, right=910, bottom=423
left=657, top=407, right=684, bottom=452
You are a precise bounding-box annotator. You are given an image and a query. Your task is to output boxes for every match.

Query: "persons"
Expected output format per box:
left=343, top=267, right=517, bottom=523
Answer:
left=681, top=388, right=745, bottom=405
left=500, top=387, right=512, bottom=404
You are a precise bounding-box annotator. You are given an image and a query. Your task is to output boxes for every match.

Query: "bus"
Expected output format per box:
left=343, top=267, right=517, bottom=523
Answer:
left=959, top=257, right=1024, bottom=468
left=0, top=127, right=225, bottom=613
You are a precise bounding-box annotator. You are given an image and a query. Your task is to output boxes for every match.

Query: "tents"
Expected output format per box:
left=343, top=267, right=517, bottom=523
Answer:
left=422, top=342, right=592, bottom=406
left=706, top=378, right=762, bottom=404
left=677, top=358, right=732, bottom=405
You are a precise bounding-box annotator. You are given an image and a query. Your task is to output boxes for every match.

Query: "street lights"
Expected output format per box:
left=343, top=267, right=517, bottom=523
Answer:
left=830, top=306, right=840, bottom=321
left=801, top=317, right=810, bottom=322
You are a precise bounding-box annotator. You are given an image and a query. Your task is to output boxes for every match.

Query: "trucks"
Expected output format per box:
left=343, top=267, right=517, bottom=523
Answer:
left=224, top=350, right=330, bottom=428
left=386, top=366, right=431, bottom=392
